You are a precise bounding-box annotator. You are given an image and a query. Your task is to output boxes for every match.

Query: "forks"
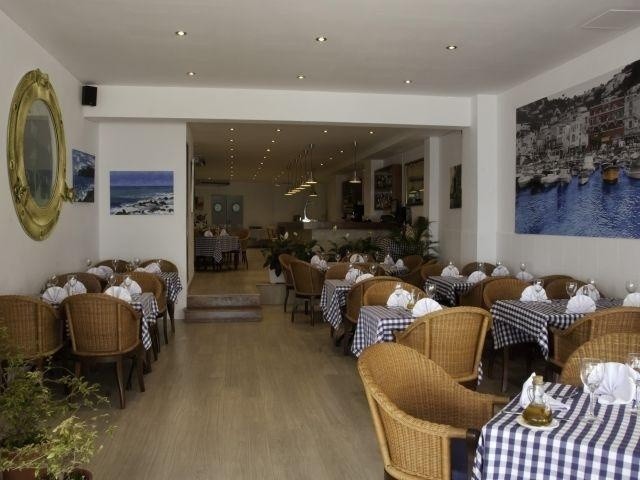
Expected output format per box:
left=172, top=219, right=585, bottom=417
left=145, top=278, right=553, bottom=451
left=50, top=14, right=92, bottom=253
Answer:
left=547, top=390, right=578, bottom=398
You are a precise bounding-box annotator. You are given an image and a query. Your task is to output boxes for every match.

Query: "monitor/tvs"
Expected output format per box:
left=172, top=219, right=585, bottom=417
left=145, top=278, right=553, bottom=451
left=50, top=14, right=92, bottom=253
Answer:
left=390, top=199, right=399, bottom=221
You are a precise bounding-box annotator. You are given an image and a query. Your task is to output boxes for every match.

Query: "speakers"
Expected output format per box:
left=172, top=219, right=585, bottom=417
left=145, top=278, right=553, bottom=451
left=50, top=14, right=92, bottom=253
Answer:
left=82, top=86, right=97, bottom=106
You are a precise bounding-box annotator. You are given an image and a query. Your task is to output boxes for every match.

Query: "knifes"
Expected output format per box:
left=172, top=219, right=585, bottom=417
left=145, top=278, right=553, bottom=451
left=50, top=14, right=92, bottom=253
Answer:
left=503, top=410, right=573, bottom=421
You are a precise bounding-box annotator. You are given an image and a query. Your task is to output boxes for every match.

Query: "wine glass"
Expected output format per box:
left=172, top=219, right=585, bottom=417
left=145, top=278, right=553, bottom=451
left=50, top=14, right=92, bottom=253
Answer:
left=532, top=278, right=639, bottom=308
left=626, top=352, right=639, bottom=416
left=392, top=278, right=438, bottom=314
left=447, top=257, right=527, bottom=282
left=320, top=250, right=392, bottom=284
left=45, top=254, right=163, bottom=302
left=578, top=357, right=605, bottom=423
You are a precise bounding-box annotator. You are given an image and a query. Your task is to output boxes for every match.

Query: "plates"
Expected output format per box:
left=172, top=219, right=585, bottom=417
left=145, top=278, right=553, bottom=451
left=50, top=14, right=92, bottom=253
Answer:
left=516, top=415, right=559, bottom=432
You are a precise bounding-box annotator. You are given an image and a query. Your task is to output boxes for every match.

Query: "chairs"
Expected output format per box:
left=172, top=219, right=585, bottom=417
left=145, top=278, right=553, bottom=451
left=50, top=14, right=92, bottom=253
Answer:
left=1, top=257, right=184, bottom=411
left=280, top=253, right=640, bottom=391
left=194, top=225, right=285, bottom=274
left=358, top=331, right=640, bottom=479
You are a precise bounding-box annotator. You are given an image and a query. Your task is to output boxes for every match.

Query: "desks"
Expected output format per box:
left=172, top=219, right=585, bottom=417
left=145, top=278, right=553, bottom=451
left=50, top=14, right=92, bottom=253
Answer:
left=372, top=236, right=430, bottom=262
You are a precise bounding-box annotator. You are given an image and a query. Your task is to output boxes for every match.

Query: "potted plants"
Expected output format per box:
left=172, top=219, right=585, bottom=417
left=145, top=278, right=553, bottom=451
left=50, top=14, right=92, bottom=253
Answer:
left=0, top=323, right=122, bottom=480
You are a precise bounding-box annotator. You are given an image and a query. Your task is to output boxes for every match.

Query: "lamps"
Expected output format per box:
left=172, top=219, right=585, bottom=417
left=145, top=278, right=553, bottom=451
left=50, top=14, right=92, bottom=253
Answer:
left=283, top=134, right=363, bottom=223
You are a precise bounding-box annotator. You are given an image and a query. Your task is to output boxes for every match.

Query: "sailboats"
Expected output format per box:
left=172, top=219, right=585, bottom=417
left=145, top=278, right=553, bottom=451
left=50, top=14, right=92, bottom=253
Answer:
left=516, top=132, right=640, bottom=191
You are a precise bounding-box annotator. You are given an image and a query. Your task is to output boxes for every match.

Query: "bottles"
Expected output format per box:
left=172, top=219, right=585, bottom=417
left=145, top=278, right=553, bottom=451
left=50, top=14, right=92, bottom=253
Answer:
left=522, top=376, right=553, bottom=428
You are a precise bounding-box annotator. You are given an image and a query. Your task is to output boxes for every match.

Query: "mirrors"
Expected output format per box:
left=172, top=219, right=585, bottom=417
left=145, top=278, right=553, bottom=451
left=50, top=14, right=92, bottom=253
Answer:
left=6, top=67, right=69, bottom=243
